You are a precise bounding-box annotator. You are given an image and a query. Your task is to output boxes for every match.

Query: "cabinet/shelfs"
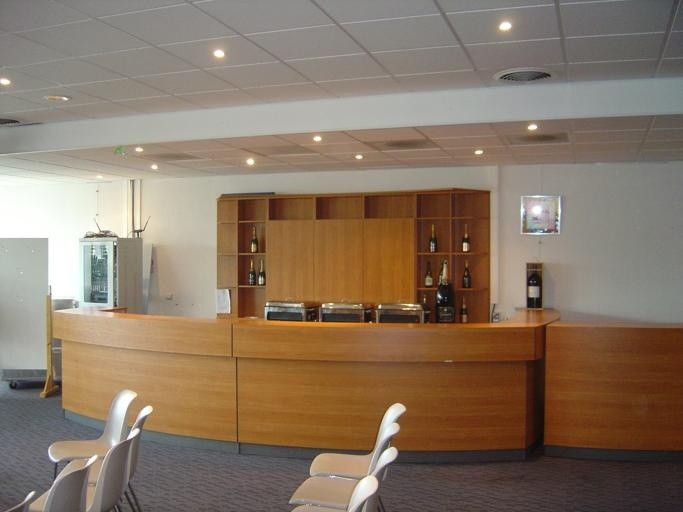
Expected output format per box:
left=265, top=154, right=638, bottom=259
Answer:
left=218, top=188, right=490, bottom=323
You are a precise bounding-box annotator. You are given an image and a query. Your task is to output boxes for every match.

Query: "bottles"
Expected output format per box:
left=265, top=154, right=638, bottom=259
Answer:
left=435, top=260, right=455, bottom=323
left=460, top=296, right=468, bottom=323
left=423, top=260, right=434, bottom=287
left=437, top=257, right=445, bottom=285
left=461, top=259, right=472, bottom=287
left=461, top=223, right=470, bottom=252
left=247, top=258, right=256, bottom=286
left=429, top=223, right=438, bottom=253
left=249, top=225, right=259, bottom=253
left=257, top=258, right=266, bottom=285
left=421, top=294, right=431, bottom=323
left=526, top=265, right=542, bottom=310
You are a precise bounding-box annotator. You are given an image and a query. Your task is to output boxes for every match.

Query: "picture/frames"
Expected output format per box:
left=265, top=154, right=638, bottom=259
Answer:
left=520, top=195, right=562, bottom=236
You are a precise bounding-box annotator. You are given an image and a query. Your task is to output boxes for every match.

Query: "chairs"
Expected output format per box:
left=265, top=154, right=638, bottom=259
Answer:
left=288, top=402, right=407, bottom=511
left=3, top=389, right=153, bottom=512
